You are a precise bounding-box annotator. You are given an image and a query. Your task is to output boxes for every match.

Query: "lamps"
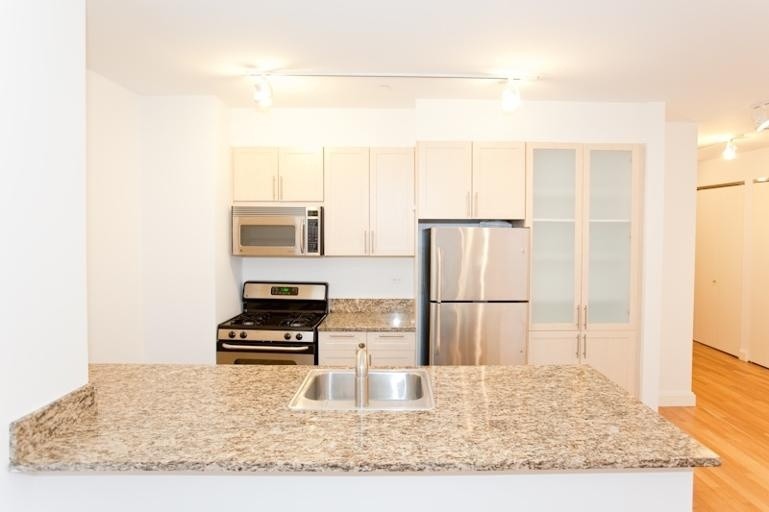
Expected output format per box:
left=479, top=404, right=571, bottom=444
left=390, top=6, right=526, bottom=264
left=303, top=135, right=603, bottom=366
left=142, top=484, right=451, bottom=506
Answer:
left=721, top=120, right=769, bottom=160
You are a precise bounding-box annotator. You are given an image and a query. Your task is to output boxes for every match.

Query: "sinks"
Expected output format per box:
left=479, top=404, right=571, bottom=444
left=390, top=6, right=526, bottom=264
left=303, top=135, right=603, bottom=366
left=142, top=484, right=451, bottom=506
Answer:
left=288, top=369, right=436, bottom=412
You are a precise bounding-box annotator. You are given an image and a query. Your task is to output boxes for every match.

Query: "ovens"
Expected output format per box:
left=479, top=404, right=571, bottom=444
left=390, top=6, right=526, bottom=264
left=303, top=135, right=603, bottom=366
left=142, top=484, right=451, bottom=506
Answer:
left=215, top=340, right=318, bottom=366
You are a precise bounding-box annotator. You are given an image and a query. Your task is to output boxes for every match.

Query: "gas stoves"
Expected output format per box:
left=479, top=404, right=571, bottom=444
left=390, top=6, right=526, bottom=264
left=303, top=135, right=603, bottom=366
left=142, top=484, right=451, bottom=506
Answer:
left=216, top=311, right=327, bottom=343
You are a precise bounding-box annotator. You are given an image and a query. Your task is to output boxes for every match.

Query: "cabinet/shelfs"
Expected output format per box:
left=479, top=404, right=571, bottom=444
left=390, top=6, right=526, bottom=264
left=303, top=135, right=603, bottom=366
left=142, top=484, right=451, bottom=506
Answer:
left=317, top=313, right=418, bottom=368
left=526, top=139, right=647, bottom=406
left=229, top=145, right=325, bottom=256
left=416, top=140, right=526, bottom=221
left=324, top=148, right=418, bottom=257
left=694, top=128, right=769, bottom=370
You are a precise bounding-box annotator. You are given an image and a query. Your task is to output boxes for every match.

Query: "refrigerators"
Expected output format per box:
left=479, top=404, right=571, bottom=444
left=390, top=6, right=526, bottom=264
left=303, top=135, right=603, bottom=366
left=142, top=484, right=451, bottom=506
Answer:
left=428, top=225, right=531, bottom=366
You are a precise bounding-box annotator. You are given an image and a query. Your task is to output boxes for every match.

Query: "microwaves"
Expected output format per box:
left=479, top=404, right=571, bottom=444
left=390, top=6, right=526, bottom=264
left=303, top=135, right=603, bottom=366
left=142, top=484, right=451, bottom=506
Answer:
left=231, top=205, right=324, bottom=258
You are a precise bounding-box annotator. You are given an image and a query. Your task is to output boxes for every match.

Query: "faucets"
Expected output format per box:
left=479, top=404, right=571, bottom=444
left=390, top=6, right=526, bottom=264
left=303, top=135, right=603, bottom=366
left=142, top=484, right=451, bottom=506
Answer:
left=356, top=344, right=370, bottom=408
